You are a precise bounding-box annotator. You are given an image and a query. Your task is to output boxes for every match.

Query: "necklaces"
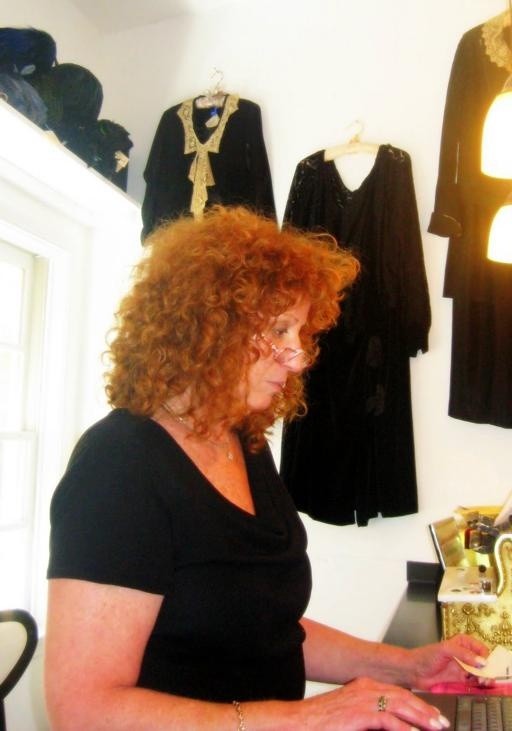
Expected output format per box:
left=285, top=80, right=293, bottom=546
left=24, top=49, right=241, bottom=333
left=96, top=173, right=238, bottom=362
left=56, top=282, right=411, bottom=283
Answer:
left=172, top=419, right=235, bottom=461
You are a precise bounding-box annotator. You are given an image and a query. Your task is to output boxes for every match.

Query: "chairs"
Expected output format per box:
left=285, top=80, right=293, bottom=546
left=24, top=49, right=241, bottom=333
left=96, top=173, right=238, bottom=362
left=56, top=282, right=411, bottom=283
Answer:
left=0, top=609, right=38, bottom=731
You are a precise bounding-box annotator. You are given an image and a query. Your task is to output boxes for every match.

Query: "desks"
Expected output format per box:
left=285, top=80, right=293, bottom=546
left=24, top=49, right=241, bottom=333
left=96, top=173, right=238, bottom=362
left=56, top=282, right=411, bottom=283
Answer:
left=382, top=561, right=457, bottom=731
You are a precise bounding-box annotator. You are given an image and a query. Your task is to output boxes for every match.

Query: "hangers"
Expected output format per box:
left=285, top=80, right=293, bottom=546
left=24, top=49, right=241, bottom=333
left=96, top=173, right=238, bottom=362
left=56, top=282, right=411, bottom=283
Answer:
left=324, top=119, right=377, bottom=161
left=196, top=71, right=226, bottom=107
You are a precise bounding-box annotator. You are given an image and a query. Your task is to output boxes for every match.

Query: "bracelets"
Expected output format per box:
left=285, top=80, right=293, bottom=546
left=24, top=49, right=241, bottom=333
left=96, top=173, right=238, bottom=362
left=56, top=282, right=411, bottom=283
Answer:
left=231, top=696, right=246, bottom=730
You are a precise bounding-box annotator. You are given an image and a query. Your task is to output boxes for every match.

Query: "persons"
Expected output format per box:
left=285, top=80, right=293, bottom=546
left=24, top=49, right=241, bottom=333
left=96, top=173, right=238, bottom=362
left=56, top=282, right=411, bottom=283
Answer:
left=41, top=203, right=495, bottom=730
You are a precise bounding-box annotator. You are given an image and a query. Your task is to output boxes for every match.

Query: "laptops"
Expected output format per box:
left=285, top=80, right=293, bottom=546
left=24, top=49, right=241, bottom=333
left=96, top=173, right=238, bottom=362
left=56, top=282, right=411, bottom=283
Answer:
left=406, top=690, right=511, bottom=731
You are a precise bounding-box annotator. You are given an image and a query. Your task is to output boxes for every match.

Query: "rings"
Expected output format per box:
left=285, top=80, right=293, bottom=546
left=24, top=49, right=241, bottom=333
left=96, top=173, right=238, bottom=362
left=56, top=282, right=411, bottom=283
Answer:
left=376, top=694, right=390, bottom=712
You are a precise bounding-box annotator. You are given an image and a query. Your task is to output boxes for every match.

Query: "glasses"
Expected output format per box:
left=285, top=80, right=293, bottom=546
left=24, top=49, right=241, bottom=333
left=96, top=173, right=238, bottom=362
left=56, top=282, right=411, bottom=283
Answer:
left=256, top=326, right=317, bottom=378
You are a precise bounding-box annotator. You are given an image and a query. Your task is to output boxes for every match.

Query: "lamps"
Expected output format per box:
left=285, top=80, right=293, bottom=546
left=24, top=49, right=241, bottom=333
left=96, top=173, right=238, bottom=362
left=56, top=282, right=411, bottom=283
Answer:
left=480, top=76, right=512, bottom=264
